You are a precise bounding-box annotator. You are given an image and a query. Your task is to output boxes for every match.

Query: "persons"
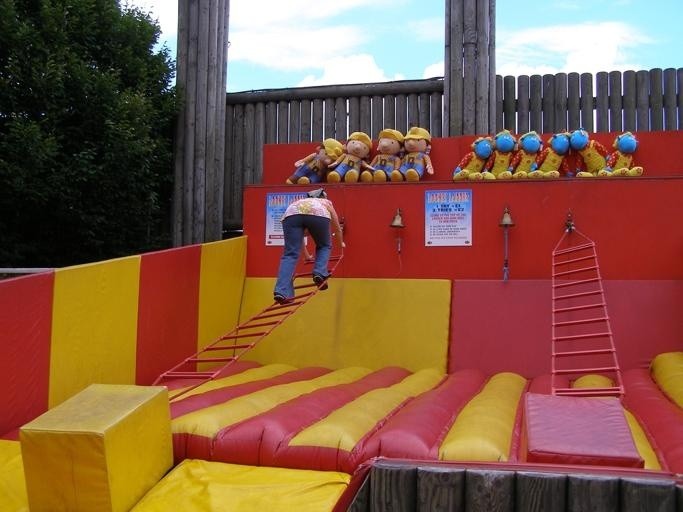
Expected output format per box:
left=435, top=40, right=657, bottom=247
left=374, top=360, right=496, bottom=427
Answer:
left=530, top=131, right=572, bottom=178
left=326, top=131, right=375, bottom=183
left=569, top=127, right=613, bottom=178
left=452, top=136, right=496, bottom=181
left=507, top=130, right=544, bottom=179
left=390, top=127, right=434, bottom=182
left=481, top=130, right=517, bottom=179
left=285, top=137, right=343, bottom=184
left=360, top=128, right=405, bottom=183
left=606, top=131, right=644, bottom=177
left=273, top=187, right=346, bottom=304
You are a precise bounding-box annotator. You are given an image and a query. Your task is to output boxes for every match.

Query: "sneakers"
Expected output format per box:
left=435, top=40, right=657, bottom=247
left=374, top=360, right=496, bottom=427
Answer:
left=273, top=292, right=292, bottom=304
left=313, top=271, right=329, bottom=289
